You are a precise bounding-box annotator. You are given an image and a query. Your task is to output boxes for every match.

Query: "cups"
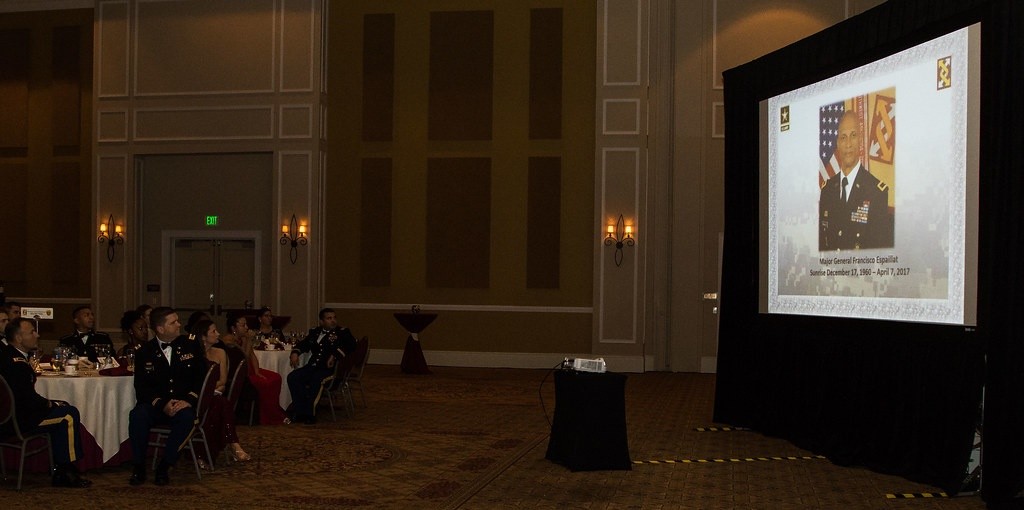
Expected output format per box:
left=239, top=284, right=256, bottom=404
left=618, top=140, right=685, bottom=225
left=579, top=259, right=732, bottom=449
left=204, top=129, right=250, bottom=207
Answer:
left=254, top=334, right=261, bottom=346
left=64, top=365, right=76, bottom=373
left=300, top=333, right=305, bottom=340
left=264, top=344, right=275, bottom=350
left=79, top=357, right=88, bottom=364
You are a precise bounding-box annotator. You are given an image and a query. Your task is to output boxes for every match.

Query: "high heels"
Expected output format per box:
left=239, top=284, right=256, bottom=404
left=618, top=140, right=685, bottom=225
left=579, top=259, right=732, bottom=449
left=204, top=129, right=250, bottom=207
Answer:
left=228, top=444, right=249, bottom=463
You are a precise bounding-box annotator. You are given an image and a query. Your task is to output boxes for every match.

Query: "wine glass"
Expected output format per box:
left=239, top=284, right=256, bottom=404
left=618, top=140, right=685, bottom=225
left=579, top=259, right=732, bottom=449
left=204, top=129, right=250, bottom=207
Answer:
left=33, top=349, right=44, bottom=373
left=290, top=333, right=296, bottom=347
left=127, top=349, right=135, bottom=372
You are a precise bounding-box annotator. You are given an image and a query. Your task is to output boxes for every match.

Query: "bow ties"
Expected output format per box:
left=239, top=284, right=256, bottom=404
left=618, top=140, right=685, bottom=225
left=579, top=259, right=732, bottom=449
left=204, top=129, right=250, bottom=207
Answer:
left=80, top=331, right=93, bottom=338
left=162, top=342, right=174, bottom=350
left=322, top=330, right=328, bottom=335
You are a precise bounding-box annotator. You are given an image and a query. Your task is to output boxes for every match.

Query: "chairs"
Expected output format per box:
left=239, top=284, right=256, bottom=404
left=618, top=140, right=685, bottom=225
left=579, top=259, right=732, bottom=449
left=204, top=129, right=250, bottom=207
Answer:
left=147, top=364, right=219, bottom=481
left=313, top=336, right=371, bottom=422
left=0, top=376, right=67, bottom=491
left=225, top=358, right=258, bottom=466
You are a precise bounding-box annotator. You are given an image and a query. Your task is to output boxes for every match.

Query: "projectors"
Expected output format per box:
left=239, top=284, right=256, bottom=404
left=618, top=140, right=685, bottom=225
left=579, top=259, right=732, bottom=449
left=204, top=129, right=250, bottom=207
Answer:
left=563, top=357, right=607, bottom=374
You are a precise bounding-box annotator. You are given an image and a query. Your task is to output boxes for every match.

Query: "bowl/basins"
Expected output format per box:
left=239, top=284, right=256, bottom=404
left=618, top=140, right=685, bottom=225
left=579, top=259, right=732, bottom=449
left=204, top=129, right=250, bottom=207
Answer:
left=97, top=357, right=107, bottom=363
left=67, top=360, right=79, bottom=365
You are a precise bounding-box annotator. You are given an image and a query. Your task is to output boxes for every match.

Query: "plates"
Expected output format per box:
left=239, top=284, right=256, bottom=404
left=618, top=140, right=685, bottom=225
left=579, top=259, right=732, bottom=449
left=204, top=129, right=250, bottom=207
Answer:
left=39, top=362, right=51, bottom=369
left=62, top=372, right=81, bottom=377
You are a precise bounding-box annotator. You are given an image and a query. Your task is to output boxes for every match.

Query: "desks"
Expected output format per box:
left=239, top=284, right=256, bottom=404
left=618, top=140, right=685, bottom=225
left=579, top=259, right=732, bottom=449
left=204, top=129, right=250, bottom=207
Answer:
left=392, top=312, right=439, bottom=374
left=544, top=369, right=633, bottom=471
left=1, top=363, right=170, bottom=469
left=254, top=350, right=312, bottom=412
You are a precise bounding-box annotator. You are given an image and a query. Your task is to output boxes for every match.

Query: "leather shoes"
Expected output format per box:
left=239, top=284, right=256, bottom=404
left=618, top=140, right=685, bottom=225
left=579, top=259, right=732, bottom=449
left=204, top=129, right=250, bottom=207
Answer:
left=155, top=467, right=167, bottom=486
left=129, top=468, right=145, bottom=485
left=52, top=473, right=91, bottom=487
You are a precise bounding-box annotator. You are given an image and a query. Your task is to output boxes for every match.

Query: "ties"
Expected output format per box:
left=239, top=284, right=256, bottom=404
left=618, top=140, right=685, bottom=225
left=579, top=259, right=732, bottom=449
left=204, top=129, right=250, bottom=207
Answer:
left=841, top=177, right=849, bottom=207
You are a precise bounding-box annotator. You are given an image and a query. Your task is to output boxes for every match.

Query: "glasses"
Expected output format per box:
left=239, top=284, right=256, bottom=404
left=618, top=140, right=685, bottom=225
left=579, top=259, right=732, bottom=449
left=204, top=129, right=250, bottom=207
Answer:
left=263, top=314, right=274, bottom=318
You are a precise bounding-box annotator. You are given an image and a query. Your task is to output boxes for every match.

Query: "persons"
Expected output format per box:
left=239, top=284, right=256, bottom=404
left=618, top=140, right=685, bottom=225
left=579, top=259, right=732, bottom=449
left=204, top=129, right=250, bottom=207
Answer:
left=0, top=318, right=92, bottom=488
left=185, top=312, right=252, bottom=470
left=222, top=312, right=291, bottom=425
left=0, top=302, right=22, bottom=339
left=252, top=306, right=286, bottom=342
left=819, top=110, right=889, bottom=249
left=129, top=307, right=206, bottom=484
left=288, top=308, right=357, bottom=424
left=59, top=306, right=116, bottom=359
left=118, top=304, right=155, bottom=356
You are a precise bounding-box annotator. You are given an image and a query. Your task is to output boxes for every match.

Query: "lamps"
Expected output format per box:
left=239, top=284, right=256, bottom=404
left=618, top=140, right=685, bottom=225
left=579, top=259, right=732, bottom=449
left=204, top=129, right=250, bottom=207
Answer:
left=98, top=214, right=124, bottom=263
left=280, top=213, right=307, bottom=264
left=604, top=214, right=635, bottom=268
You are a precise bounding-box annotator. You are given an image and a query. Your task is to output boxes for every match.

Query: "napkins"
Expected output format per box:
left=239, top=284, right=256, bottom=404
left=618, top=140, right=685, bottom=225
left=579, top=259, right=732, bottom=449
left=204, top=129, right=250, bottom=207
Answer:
left=254, top=341, right=284, bottom=353
left=98, top=358, right=134, bottom=376
left=40, top=354, right=64, bottom=372
